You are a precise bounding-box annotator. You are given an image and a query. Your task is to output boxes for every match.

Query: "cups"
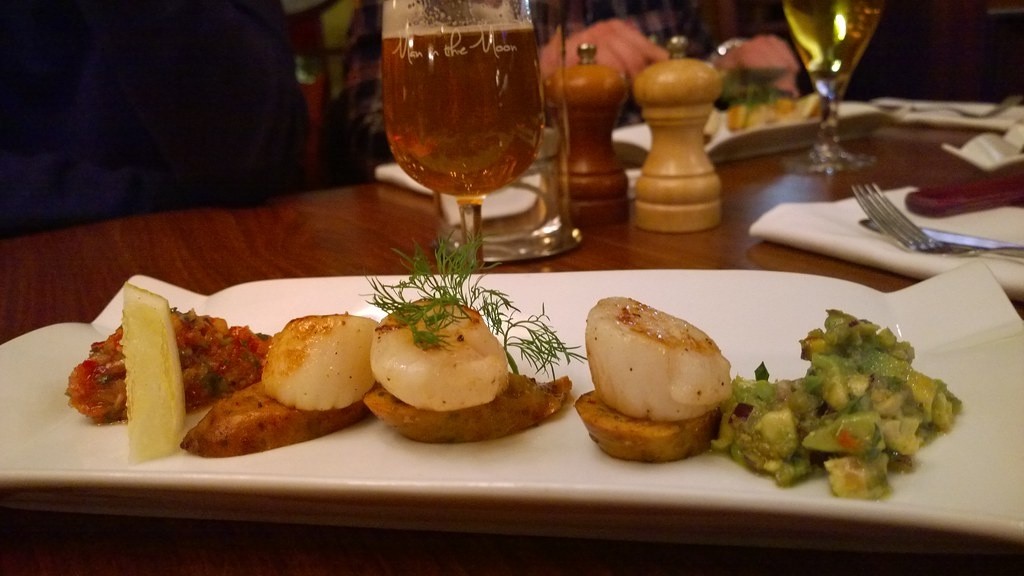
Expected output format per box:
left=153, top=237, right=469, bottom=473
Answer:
left=434, top=0, right=578, bottom=260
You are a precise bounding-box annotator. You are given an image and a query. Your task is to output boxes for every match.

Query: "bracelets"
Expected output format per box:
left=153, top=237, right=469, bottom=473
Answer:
left=702, top=36, right=744, bottom=67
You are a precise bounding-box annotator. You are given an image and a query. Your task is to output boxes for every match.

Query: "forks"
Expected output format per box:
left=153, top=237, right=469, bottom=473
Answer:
left=851, top=182, right=1024, bottom=255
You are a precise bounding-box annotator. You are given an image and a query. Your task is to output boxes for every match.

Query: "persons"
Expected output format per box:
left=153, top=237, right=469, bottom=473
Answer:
left=321, top=0, right=799, bottom=192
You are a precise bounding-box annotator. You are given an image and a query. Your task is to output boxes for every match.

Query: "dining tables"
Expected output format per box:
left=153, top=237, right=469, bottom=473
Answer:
left=0, top=116, right=1024, bottom=576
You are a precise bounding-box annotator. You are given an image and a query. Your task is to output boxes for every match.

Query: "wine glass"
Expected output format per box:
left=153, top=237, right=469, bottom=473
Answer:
left=779, top=0, right=884, bottom=173
left=382, top=0, right=543, bottom=274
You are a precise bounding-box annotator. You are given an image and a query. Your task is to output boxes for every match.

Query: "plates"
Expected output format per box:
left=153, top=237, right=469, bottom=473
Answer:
left=0, top=261, right=1024, bottom=544
left=611, top=100, right=889, bottom=163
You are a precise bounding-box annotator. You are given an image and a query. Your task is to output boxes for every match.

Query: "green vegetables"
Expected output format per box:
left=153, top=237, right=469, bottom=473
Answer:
left=367, top=229, right=588, bottom=385
left=723, top=71, right=779, bottom=106
